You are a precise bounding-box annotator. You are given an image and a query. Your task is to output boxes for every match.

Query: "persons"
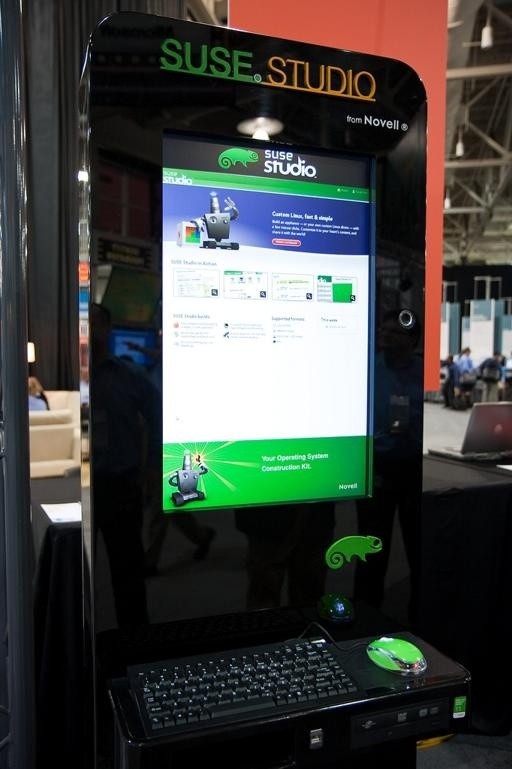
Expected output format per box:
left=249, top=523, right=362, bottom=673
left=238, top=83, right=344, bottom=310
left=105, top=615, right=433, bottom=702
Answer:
left=441, top=347, right=507, bottom=410
left=28, top=376, right=49, bottom=410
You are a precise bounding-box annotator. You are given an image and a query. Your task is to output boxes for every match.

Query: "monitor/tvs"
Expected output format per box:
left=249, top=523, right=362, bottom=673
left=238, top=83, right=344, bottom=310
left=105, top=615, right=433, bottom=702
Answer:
left=162, top=131, right=381, bottom=513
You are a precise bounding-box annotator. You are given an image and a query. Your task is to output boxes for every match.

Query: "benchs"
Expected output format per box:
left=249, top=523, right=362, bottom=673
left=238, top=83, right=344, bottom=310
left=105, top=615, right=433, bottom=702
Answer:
left=27, top=388, right=83, bottom=478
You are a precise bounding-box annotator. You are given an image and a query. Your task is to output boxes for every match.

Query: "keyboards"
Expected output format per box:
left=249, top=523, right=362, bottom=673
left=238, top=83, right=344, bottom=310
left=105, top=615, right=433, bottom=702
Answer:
left=130, top=635, right=360, bottom=742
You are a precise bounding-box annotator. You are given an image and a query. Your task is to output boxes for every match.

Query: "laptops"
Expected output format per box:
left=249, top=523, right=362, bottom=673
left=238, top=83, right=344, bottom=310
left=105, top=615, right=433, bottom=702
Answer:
left=429, top=401, right=511, bottom=458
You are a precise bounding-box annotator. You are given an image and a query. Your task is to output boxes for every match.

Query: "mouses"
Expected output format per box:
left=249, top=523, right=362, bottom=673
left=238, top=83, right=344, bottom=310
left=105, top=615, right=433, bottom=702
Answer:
left=322, top=590, right=356, bottom=625
left=364, top=636, right=429, bottom=679
